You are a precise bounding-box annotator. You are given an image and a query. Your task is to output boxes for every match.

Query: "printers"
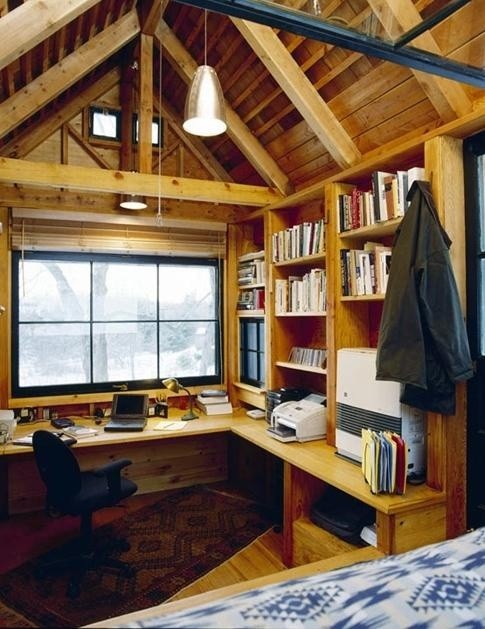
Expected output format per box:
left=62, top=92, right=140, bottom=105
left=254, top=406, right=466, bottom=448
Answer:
left=266, top=393, right=327, bottom=443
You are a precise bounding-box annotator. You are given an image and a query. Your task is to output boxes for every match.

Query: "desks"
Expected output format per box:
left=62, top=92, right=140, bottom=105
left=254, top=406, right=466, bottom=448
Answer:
left=2, top=401, right=447, bottom=558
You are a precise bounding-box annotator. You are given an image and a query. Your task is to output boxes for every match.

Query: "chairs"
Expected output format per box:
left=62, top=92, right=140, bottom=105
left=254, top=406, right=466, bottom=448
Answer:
left=28, top=429, right=140, bottom=596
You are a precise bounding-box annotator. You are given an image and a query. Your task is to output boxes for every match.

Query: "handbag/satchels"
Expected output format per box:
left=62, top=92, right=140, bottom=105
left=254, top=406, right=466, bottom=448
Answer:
left=309, top=487, right=376, bottom=546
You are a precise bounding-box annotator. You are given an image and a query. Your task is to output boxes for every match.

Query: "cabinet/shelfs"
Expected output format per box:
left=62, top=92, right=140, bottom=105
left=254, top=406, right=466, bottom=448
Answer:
left=222, top=135, right=451, bottom=489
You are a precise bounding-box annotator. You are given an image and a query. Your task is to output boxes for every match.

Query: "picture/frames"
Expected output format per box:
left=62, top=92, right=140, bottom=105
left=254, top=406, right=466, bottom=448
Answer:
left=374, top=245, right=393, bottom=296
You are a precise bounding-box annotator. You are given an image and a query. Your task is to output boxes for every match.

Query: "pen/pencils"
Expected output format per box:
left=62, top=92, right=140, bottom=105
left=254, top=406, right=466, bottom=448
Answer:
left=158, top=393, right=168, bottom=402
left=164, top=422, right=174, bottom=428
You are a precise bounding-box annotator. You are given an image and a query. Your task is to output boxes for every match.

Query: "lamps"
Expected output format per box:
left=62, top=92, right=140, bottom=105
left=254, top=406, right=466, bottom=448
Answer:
left=177, top=7, right=227, bottom=140
left=118, top=192, right=149, bottom=213
left=162, top=377, right=199, bottom=421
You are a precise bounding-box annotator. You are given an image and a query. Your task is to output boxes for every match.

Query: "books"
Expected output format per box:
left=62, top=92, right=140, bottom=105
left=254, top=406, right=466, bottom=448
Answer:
left=201, top=388, right=226, bottom=397
left=154, top=420, right=188, bottom=431
left=197, top=394, right=229, bottom=405
left=195, top=401, right=233, bottom=415
left=237, top=166, right=429, bottom=370
left=362, top=427, right=409, bottom=496
left=13, top=424, right=99, bottom=446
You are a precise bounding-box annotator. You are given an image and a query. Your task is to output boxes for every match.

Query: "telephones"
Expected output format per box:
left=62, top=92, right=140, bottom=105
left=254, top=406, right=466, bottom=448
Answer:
left=50, top=418, right=74, bottom=428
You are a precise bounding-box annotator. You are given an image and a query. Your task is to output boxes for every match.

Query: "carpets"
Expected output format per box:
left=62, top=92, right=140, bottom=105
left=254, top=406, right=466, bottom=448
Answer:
left=0, top=482, right=282, bottom=629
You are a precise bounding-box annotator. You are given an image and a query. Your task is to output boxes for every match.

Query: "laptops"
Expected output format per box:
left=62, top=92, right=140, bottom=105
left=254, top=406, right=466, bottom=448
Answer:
left=104, top=394, right=149, bottom=431
